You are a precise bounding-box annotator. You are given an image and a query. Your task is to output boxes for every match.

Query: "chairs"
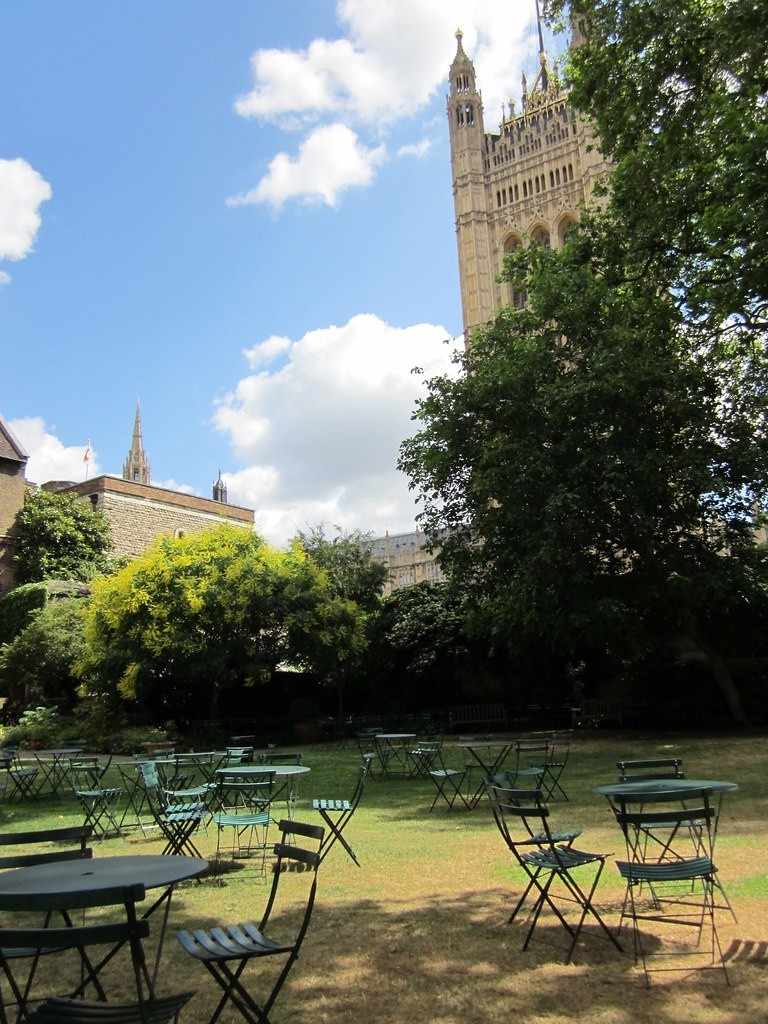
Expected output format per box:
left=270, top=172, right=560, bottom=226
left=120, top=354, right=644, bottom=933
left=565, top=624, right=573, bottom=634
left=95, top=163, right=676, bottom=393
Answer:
left=0, top=715, right=467, bottom=885
left=482, top=770, right=583, bottom=933
left=492, top=785, right=624, bottom=965
left=614, top=786, right=731, bottom=985
left=530, top=731, right=574, bottom=801
left=0, top=824, right=95, bottom=1024
left=459, top=736, right=499, bottom=801
left=502, top=737, right=550, bottom=807
left=0, top=883, right=199, bottom=1024
left=175, top=821, right=325, bottom=1024
left=619, top=760, right=709, bottom=907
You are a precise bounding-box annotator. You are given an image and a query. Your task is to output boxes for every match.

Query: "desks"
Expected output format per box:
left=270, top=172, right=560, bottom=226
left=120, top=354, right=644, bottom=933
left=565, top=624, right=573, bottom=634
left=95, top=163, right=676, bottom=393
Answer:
left=459, top=741, right=518, bottom=808
left=32, top=749, right=84, bottom=802
left=0, top=854, right=210, bottom=1024
left=374, top=733, right=416, bottom=777
left=113, top=760, right=181, bottom=840
left=191, top=750, right=230, bottom=807
left=591, top=779, right=740, bottom=934
left=214, top=766, right=311, bottom=859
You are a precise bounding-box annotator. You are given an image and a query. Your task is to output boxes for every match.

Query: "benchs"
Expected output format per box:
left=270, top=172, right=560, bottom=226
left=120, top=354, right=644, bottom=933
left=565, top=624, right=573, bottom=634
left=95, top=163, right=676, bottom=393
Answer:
left=571, top=698, right=624, bottom=733
left=448, top=702, right=507, bottom=735
